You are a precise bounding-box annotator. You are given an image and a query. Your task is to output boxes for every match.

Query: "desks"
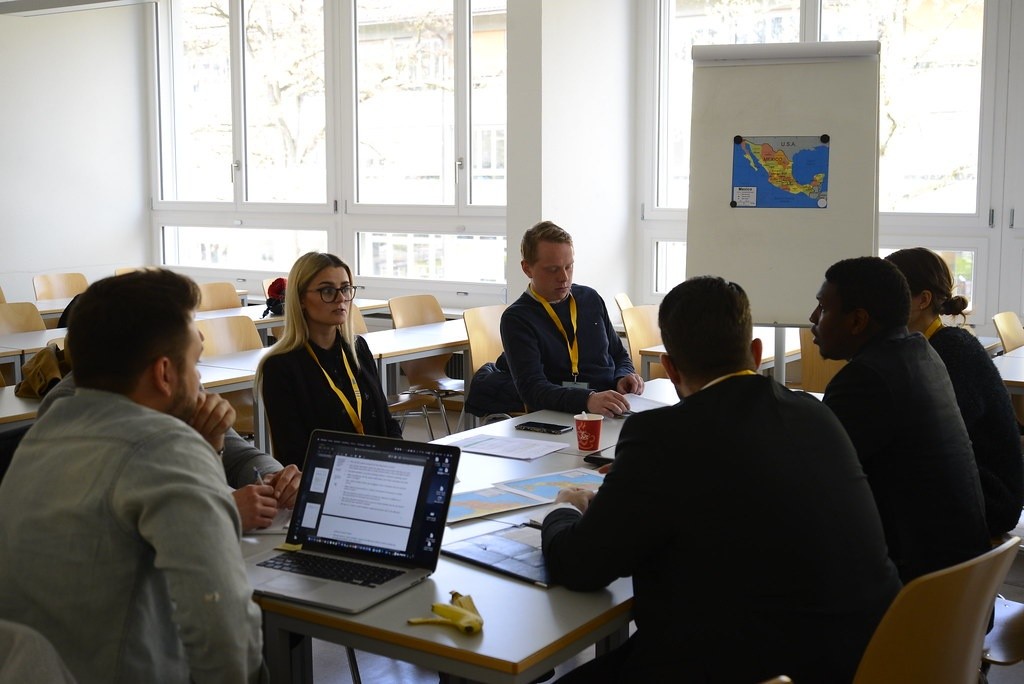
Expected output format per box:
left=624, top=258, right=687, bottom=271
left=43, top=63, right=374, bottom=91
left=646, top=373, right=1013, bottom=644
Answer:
left=363, top=319, right=477, bottom=430
left=977, top=337, right=1003, bottom=356
left=0, top=317, right=68, bottom=365
left=196, top=336, right=380, bottom=375
left=638, top=327, right=802, bottom=382
left=0, top=347, right=266, bottom=456
left=239, top=377, right=826, bottom=684
left=14, top=290, right=248, bottom=320
left=194, top=298, right=390, bottom=347
left=992, top=344, right=1024, bottom=395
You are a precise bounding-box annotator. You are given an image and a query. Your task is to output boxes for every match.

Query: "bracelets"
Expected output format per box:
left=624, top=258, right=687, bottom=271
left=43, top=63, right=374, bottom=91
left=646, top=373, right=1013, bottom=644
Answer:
left=218, top=447, right=224, bottom=455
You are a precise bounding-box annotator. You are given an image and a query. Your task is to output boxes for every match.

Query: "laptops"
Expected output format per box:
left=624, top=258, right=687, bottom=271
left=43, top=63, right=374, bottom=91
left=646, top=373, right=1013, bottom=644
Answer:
left=245, top=430, right=460, bottom=614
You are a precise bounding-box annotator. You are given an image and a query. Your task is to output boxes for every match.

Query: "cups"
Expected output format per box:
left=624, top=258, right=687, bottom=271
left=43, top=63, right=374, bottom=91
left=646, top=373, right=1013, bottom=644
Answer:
left=573, top=414, right=604, bottom=452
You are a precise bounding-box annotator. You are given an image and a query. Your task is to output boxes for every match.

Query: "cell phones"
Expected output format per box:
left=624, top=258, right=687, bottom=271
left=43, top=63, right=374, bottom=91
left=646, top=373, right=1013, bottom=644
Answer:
left=515, top=422, right=573, bottom=435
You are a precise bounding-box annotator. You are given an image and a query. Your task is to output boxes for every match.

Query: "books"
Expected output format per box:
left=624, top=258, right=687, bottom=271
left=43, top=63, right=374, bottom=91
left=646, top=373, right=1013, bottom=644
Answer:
left=229, top=486, right=292, bottom=534
left=620, top=378, right=680, bottom=416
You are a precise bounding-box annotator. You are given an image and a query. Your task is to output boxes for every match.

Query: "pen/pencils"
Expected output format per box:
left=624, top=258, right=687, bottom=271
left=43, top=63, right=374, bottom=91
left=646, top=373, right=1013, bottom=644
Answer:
left=253, top=466, right=264, bottom=485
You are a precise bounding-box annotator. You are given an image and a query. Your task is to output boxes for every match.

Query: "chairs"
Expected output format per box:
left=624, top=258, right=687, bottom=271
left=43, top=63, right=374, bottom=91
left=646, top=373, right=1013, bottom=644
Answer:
left=0, top=270, right=1024, bottom=684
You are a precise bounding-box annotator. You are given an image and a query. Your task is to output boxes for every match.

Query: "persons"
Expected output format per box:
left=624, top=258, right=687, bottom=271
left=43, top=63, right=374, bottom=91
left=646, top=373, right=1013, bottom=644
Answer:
left=881, top=248, right=1024, bottom=536
left=253, top=250, right=554, bottom=684
left=809, top=255, right=991, bottom=582
left=0, top=267, right=265, bottom=684
left=542, top=276, right=902, bottom=684
left=500, top=219, right=645, bottom=417
left=36, top=340, right=302, bottom=529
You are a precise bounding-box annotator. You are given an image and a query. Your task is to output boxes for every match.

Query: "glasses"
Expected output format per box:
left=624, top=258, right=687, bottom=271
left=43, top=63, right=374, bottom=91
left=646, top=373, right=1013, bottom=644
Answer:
left=305, top=285, right=357, bottom=303
